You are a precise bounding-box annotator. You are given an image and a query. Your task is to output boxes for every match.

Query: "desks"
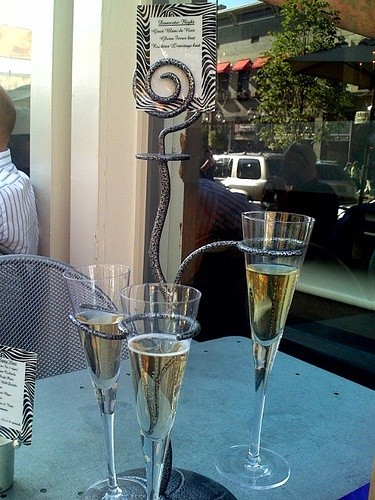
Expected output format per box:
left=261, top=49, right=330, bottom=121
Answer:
left=0, top=336, right=375, bottom=500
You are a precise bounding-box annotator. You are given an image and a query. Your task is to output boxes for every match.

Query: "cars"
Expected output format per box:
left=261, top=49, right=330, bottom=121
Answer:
left=211, top=153, right=289, bottom=205
left=311, top=160, right=358, bottom=200
left=337, top=197, right=375, bottom=238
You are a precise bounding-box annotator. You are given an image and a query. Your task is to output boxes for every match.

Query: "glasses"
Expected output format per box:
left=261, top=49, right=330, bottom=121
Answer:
left=288, top=143, right=309, bottom=165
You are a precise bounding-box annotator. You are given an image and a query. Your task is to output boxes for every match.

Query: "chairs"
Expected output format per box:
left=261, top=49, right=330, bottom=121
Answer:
left=0, top=254, right=129, bottom=380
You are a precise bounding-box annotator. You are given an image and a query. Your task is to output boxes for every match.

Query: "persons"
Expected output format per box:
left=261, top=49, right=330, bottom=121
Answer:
left=196, top=149, right=266, bottom=273
left=262, top=140, right=338, bottom=231
left=0, top=85, right=41, bottom=276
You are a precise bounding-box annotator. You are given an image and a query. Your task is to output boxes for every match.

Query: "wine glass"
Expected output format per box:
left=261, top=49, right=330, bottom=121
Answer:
left=118, top=283, right=201, bottom=500
left=63, top=264, right=148, bottom=500
left=214, top=212, right=314, bottom=491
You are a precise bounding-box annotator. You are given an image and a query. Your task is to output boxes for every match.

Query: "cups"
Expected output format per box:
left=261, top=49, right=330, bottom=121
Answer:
left=0, top=436, right=14, bottom=492
